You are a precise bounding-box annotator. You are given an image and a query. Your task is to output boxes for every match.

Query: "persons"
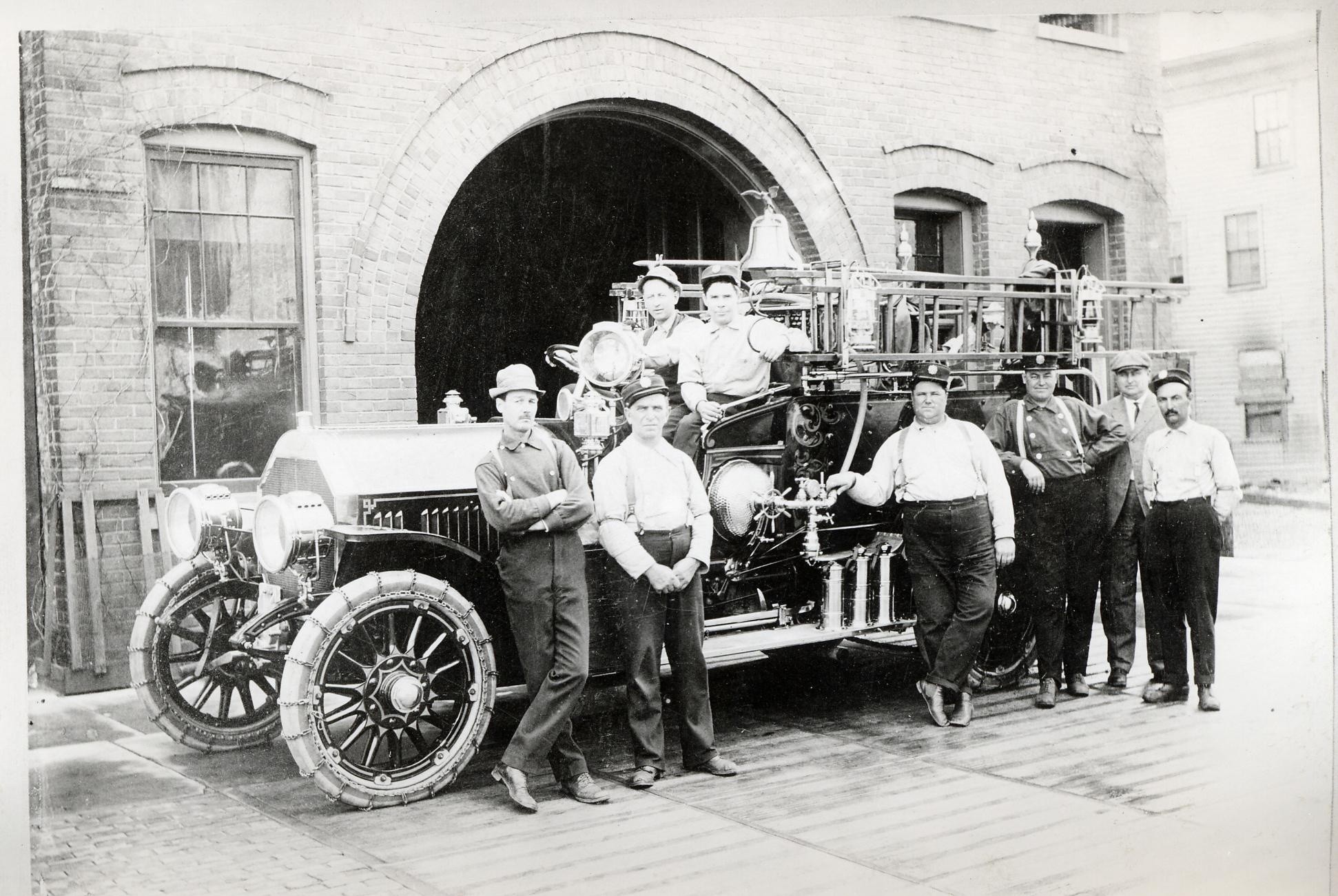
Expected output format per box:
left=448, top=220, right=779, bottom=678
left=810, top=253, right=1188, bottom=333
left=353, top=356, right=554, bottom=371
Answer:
left=1139, top=367, right=1243, bottom=712
left=673, top=264, right=814, bottom=463
left=1095, top=350, right=1165, bottom=688
left=544, top=266, right=705, bottom=463
left=982, top=354, right=1128, bottom=709
left=475, top=363, right=610, bottom=812
left=591, top=375, right=739, bottom=787
left=826, top=362, right=1015, bottom=726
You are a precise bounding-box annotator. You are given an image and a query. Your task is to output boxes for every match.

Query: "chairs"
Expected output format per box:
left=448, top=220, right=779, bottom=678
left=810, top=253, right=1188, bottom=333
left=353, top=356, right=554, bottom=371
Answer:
left=694, top=350, right=793, bottom=454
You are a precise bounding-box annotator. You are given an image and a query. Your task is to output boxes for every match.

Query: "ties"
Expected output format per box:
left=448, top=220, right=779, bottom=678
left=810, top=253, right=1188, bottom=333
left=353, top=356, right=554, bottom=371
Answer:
left=1133, top=402, right=1140, bottom=421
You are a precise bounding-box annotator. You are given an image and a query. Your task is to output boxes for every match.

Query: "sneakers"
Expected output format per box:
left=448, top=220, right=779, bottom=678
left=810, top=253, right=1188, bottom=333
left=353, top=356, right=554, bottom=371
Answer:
left=1036, top=678, right=1058, bottom=706
left=1065, top=672, right=1089, bottom=696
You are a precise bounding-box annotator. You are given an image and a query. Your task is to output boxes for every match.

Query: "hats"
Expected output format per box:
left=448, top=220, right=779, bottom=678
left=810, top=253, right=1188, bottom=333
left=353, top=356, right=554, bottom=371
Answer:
left=621, top=375, right=669, bottom=408
left=638, top=266, right=683, bottom=295
left=489, top=364, right=546, bottom=399
left=1022, top=355, right=1059, bottom=370
left=1110, top=349, right=1151, bottom=372
left=700, top=264, right=742, bottom=287
left=915, top=362, right=950, bottom=382
left=1149, top=368, right=1192, bottom=393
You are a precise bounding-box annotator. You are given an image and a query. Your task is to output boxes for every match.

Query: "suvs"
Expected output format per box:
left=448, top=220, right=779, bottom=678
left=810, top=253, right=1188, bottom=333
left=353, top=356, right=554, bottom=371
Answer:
left=125, top=258, right=1121, bottom=814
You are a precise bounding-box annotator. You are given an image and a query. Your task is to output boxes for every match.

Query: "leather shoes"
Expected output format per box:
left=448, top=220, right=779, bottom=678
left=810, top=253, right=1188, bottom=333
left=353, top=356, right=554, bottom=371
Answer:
left=1107, top=668, right=1127, bottom=686
left=491, top=761, right=539, bottom=811
left=629, top=766, right=661, bottom=786
left=916, top=680, right=950, bottom=727
left=1142, top=683, right=1189, bottom=702
left=687, top=755, right=738, bottom=774
left=1198, top=683, right=1220, bottom=710
left=561, top=772, right=611, bottom=802
left=1154, top=671, right=1171, bottom=683
left=948, top=692, right=974, bottom=726
